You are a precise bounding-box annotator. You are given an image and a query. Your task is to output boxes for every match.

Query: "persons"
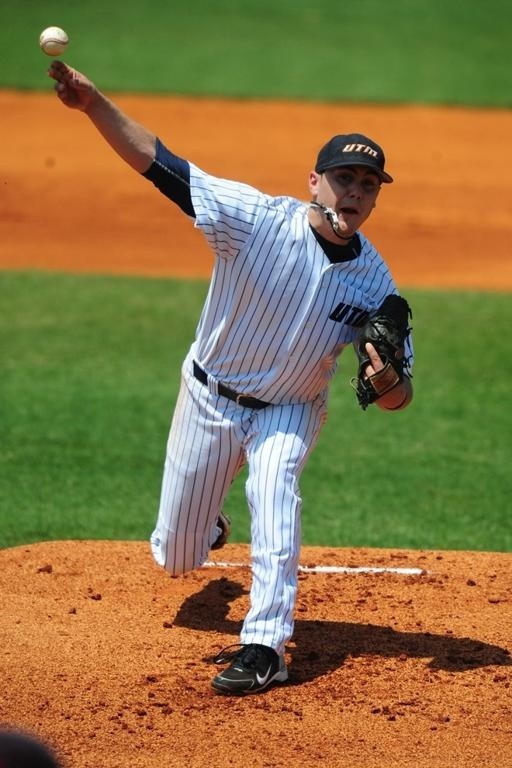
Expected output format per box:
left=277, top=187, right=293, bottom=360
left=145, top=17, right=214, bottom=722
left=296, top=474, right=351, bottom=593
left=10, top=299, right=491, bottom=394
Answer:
left=0, top=727, right=65, bottom=768
left=47, top=56, right=417, bottom=695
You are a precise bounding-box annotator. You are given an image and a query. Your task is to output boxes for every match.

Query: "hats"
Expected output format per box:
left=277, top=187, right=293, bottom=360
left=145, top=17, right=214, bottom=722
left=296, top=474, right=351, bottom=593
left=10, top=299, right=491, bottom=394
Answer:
left=313, top=132, right=394, bottom=184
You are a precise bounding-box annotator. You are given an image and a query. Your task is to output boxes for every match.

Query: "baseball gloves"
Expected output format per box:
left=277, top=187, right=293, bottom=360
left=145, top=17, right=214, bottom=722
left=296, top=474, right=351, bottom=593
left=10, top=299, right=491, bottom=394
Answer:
left=349, top=293, right=411, bottom=408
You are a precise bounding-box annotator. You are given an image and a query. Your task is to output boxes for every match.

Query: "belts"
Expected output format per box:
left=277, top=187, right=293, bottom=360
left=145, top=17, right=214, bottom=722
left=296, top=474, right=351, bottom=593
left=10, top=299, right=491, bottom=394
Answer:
left=191, top=358, right=276, bottom=412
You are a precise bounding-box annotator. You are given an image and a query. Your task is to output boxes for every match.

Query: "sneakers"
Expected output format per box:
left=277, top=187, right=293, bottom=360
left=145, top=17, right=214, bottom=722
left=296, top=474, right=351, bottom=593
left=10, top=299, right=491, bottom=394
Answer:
left=207, top=641, right=290, bottom=697
left=209, top=513, right=233, bottom=551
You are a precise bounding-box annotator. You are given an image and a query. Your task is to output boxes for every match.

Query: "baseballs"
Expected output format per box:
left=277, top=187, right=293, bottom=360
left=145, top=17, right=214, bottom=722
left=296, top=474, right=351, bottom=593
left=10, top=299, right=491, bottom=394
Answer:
left=39, top=27, right=68, bottom=56
